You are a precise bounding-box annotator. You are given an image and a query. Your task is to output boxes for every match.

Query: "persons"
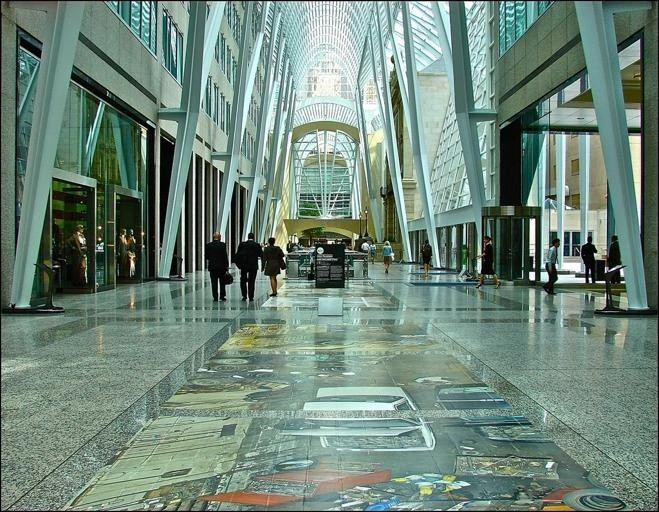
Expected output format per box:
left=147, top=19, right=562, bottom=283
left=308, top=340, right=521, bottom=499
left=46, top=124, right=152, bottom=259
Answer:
left=73, top=224, right=88, bottom=284
left=473, top=235, right=501, bottom=289
left=119, top=228, right=137, bottom=277
left=542, top=238, right=561, bottom=294
left=205, top=231, right=284, bottom=301
left=581, top=235, right=598, bottom=283
left=286, top=239, right=394, bottom=274
left=607, top=234, right=622, bottom=284
left=420, top=239, right=433, bottom=277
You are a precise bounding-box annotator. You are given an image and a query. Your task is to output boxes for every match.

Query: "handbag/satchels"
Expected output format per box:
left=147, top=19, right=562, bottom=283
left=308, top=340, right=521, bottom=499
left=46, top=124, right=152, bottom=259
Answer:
left=279, top=257, right=287, bottom=270
left=390, top=253, right=395, bottom=261
left=224, top=272, right=233, bottom=284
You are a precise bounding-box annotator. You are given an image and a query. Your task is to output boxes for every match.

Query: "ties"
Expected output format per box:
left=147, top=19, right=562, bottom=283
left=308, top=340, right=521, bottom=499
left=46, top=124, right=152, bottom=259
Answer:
left=556, top=248, right=557, bottom=260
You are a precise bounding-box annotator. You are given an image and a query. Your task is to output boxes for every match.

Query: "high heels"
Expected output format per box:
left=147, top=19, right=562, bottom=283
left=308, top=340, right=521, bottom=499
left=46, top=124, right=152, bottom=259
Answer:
left=476, top=283, right=484, bottom=289
left=270, top=293, right=277, bottom=296
left=494, top=281, right=501, bottom=289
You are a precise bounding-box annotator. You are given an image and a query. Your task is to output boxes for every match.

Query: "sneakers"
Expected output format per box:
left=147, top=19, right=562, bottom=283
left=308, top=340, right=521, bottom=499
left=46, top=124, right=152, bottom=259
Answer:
left=213, top=298, right=218, bottom=302
left=250, top=298, right=254, bottom=301
left=242, top=297, right=247, bottom=301
left=220, top=297, right=227, bottom=301
left=385, top=268, right=388, bottom=274
left=543, top=284, right=557, bottom=295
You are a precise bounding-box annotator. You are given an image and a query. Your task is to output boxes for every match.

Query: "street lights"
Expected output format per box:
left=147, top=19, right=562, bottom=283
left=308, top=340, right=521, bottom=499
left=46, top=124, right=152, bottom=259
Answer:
left=364, top=204, right=370, bottom=237
left=357, top=210, right=363, bottom=238
